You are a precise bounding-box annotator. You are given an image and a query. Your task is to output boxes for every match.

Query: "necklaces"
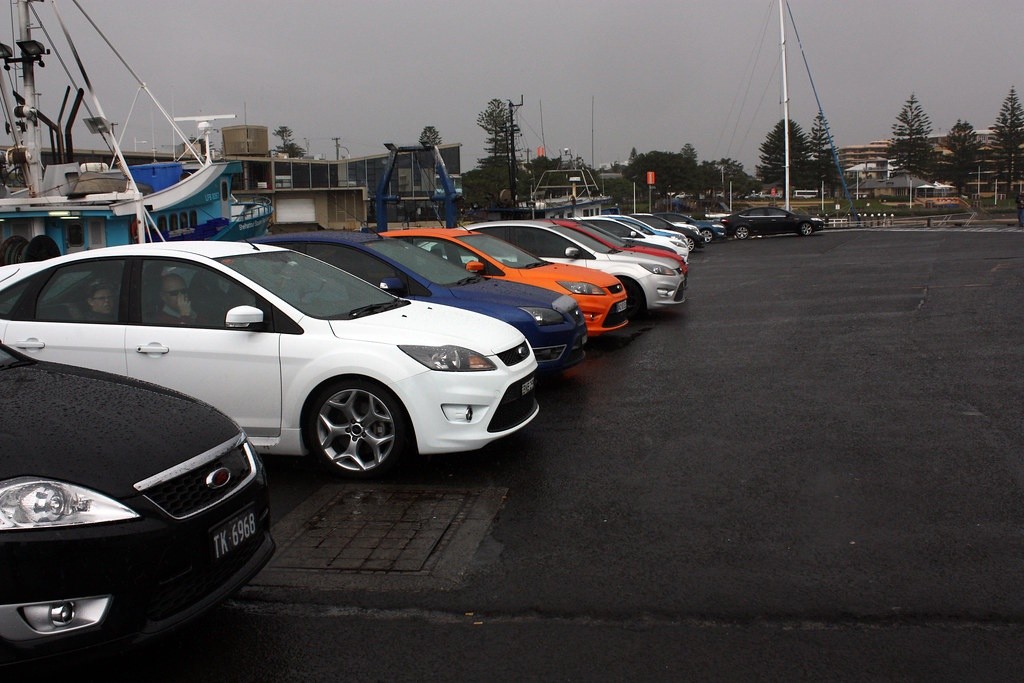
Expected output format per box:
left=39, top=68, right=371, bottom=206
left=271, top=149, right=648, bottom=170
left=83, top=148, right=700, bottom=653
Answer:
left=150, top=275, right=202, bottom=326
left=88, top=281, right=118, bottom=323
left=1015, top=190, right=1024, bottom=226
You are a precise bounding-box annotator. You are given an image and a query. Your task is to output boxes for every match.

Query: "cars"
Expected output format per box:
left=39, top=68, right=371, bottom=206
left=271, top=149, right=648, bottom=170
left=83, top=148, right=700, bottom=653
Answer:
left=652, top=213, right=728, bottom=243
left=254, top=233, right=587, bottom=375
left=540, top=220, right=688, bottom=279
left=592, top=214, right=689, bottom=247
left=720, top=207, right=824, bottom=240
left=419, top=221, right=688, bottom=322
left=628, top=214, right=705, bottom=253
left=377, top=229, right=629, bottom=337
left=566, top=217, right=689, bottom=265
left=0, top=340, right=275, bottom=672
left=0, top=240, right=541, bottom=480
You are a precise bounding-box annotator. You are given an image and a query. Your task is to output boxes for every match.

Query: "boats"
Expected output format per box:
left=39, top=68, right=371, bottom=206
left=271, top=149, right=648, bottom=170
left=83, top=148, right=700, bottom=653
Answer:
left=0, top=0, right=273, bottom=264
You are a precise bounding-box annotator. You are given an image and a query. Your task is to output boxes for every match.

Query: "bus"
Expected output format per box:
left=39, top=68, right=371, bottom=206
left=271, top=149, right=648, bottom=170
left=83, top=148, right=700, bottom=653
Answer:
left=793, top=190, right=819, bottom=199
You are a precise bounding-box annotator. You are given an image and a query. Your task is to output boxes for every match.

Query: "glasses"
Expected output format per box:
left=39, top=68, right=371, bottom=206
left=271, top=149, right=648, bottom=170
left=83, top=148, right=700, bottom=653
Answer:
left=90, top=295, right=113, bottom=300
left=162, top=289, right=188, bottom=297
